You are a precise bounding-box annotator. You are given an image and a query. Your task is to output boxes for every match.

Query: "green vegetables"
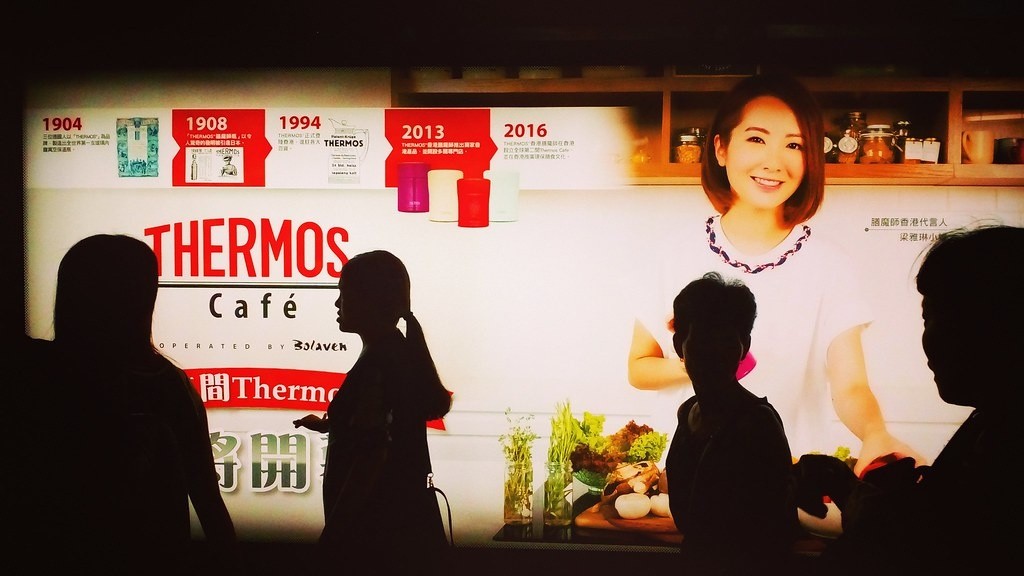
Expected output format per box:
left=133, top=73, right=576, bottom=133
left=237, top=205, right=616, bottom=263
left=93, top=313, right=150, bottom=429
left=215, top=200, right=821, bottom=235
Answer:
left=570, top=412, right=668, bottom=475
left=833, top=446, right=850, bottom=460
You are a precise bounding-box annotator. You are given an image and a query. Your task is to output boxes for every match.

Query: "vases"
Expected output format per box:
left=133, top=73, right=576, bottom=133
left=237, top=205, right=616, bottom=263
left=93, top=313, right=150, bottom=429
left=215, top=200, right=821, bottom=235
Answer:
left=544, top=459, right=575, bottom=528
left=503, top=461, right=533, bottom=527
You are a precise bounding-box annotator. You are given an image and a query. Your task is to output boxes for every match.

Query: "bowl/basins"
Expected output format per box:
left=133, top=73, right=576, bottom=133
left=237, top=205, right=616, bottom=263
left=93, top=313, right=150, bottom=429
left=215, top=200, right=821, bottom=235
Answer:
left=571, top=461, right=636, bottom=494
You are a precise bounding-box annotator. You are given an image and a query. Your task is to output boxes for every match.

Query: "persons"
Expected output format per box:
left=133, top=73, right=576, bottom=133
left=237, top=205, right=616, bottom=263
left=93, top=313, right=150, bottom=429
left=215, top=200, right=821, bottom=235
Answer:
left=666, top=272, right=803, bottom=576
left=292, top=250, right=454, bottom=576
left=791, top=224, right=1024, bottom=576
left=628, top=72, right=927, bottom=486
left=0, top=234, right=245, bottom=575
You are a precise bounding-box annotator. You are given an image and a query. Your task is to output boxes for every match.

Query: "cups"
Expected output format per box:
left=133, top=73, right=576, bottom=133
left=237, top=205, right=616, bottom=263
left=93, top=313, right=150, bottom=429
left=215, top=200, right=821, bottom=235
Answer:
left=962, top=131, right=995, bottom=164
left=543, top=460, right=573, bottom=526
left=994, top=137, right=1024, bottom=164
left=397, top=162, right=518, bottom=228
left=503, top=461, right=533, bottom=525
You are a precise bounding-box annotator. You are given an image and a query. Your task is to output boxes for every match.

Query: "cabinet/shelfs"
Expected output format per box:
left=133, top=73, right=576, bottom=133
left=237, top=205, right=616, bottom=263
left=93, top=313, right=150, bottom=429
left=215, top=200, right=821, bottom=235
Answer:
left=386, top=65, right=1024, bottom=187
